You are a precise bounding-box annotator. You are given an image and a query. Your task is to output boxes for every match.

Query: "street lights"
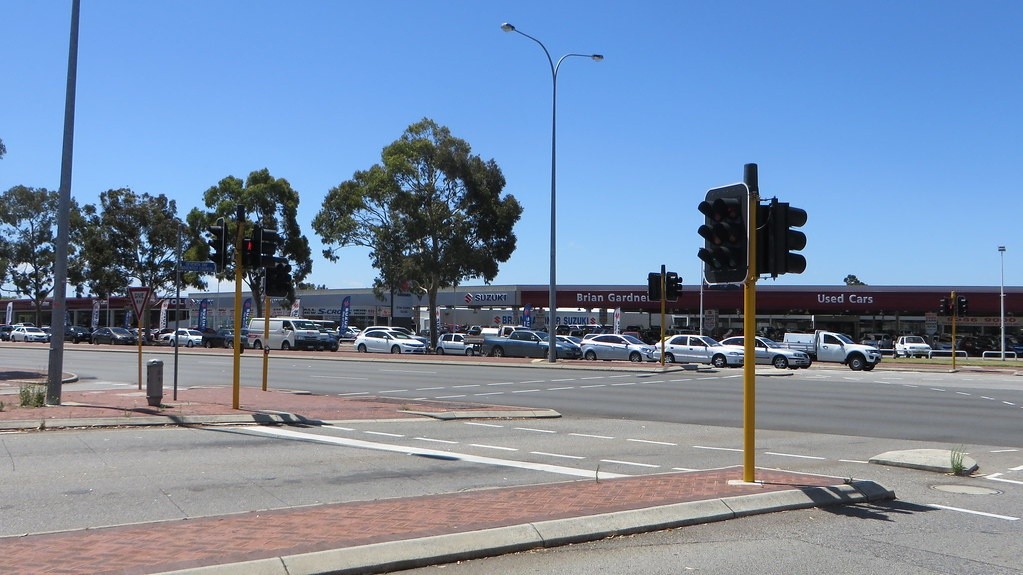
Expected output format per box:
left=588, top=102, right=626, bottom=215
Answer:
left=997, top=245, right=1007, bottom=361
left=500, top=22, right=605, bottom=363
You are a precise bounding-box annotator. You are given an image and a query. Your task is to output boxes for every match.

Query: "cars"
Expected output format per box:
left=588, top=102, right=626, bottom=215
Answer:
left=435, top=333, right=482, bottom=357
left=580, top=334, right=656, bottom=363
left=717, top=335, right=811, bottom=370
left=652, top=334, right=744, bottom=368
left=312, top=325, right=1023, bottom=358
left=353, top=329, right=427, bottom=355
left=859, top=330, right=1023, bottom=358
left=0, top=322, right=249, bottom=349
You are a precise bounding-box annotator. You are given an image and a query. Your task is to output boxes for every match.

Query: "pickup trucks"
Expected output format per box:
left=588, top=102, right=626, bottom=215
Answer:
left=775, top=329, right=882, bottom=371
left=894, top=336, right=933, bottom=359
left=484, top=330, right=582, bottom=360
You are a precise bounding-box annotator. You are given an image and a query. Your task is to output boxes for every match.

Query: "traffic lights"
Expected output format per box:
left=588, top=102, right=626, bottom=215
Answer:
left=207, top=218, right=226, bottom=273
left=758, top=202, right=806, bottom=274
left=938, top=297, right=946, bottom=316
left=253, top=222, right=278, bottom=270
left=697, top=182, right=749, bottom=286
left=665, top=272, right=683, bottom=303
left=242, top=238, right=256, bottom=269
left=647, top=272, right=662, bottom=302
left=957, top=296, right=968, bottom=317
left=265, top=257, right=292, bottom=298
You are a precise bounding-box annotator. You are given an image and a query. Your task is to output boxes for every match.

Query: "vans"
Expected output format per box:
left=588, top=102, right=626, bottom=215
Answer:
left=247, top=317, right=322, bottom=352
